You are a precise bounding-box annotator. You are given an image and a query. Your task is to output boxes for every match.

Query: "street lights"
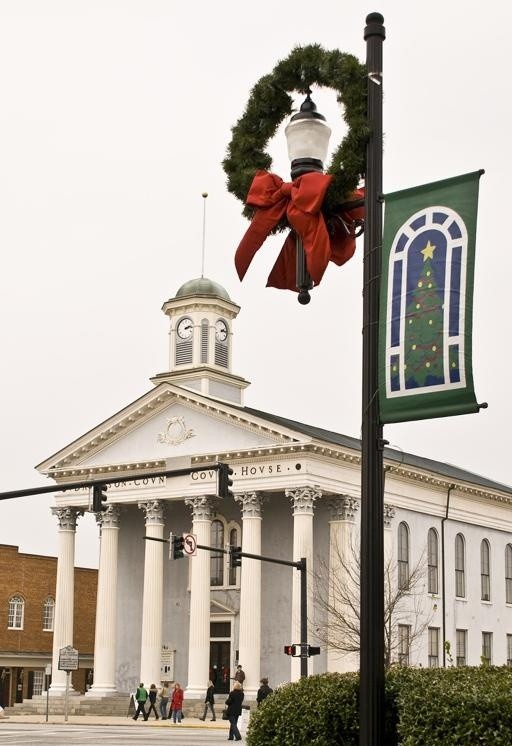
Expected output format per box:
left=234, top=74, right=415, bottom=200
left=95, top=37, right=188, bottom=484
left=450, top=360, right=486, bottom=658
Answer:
left=284, top=13, right=390, bottom=746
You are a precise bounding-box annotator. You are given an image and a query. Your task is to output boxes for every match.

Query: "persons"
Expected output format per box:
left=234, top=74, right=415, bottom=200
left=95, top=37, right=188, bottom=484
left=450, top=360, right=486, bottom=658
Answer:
left=198, top=680, right=217, bottom=722
left=131, top=682, right=149, bottom=722
left=233, top=664, right=246, bottom=692
left=170, top=681, right=184, bottom=725
left=256, top=677, right=273, bottom=708
left=145, top=683, right=160, bottom=721
left=167, top=684, right=185, bottom=720
left=225, top=681, right=245, bottom=742
left=158, top=680, right=170, bottom=720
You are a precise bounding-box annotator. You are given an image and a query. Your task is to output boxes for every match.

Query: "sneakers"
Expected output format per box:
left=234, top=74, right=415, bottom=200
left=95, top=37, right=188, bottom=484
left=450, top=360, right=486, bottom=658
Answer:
left=132, top=716, right=184, bottom=723
left=229, top=736, right=242, bottom=741
left=200, top=718, right=216, bottom=721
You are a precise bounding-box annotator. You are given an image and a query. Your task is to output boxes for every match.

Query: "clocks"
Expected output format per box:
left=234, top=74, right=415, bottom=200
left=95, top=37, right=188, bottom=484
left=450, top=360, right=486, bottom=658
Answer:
left=215, top=320, right=227, bottom=341
left=176, top=318, right=193, bottom=339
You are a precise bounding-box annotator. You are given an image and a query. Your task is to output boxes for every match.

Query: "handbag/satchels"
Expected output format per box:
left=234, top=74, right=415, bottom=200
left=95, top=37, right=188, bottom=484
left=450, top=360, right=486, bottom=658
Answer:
left=222, top=710, right=229, bottom=720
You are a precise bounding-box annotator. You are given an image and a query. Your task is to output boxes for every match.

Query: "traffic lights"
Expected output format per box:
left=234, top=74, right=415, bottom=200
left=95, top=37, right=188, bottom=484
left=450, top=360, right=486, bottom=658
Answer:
left=93, top=483, right=107, bottom=513
left=229, top=545, right=241, bottom=570
left=219, top=463, right=233, bottom=498
left=169, top=531, right=184, bottom=561
left=284, top=645, right=296, bottom=656
